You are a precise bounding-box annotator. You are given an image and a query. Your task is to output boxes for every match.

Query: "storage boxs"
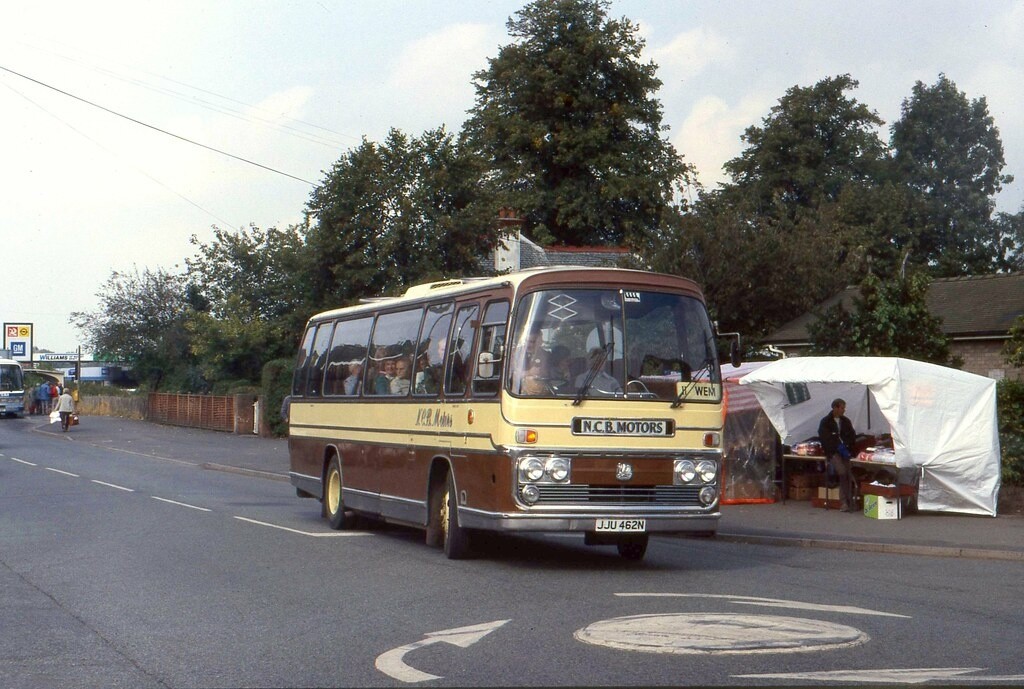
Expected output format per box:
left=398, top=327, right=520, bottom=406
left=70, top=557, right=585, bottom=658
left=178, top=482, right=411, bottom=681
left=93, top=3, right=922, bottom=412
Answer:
left=817, top=486, right=843, bottom=500
left=859, top=481, right=917, bottom=496
left=863, top=495, right=902, bottom=521
left=788, top=487, right=813, bottom=500
left=790, top=472, right=812, bottom=487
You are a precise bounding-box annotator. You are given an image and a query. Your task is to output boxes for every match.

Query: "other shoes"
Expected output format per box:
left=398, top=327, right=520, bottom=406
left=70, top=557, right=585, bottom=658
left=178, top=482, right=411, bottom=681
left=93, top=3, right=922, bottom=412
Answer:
left=840, top=503, right=849, bottom=512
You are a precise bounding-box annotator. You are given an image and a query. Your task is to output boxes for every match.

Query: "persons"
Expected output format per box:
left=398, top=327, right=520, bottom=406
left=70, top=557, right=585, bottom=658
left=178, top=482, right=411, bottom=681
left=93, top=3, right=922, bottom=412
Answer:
left=641, top=353, right=682, bottom=398
left=875, top=437, right=892, bottom=447
left=294, top=352, right=319, bottom=396
left=343, top=328, right=623, bottom=395
left=818, top=398, right=859, bottom=513
left=28, top=380, right=63, bottom=416
left=281, top=395, right=289, bottom=424
left=54, top=388, right=76, bottom=432
left=0, top=377, right=15, bottom=390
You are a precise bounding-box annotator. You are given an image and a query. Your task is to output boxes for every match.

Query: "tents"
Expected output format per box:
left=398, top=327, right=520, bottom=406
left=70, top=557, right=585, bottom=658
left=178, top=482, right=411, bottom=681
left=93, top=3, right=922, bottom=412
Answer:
left=637, top=359, right=778, bottom=505
left=738, top=355, right=1002, bottom=517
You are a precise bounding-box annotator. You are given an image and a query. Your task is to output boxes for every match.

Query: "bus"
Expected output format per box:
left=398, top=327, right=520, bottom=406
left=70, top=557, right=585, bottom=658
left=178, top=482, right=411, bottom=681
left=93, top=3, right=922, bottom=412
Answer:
left=288, top=262, right=742, bottom=563
left=0, top=358, right=25, bottom=415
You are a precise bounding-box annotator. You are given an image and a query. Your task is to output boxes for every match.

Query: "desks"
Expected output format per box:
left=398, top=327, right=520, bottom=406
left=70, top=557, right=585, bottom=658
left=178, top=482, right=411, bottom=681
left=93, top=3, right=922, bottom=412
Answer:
left=782, top=451, right=830, bottom=510
left=847, top=459, right=913, bottom=513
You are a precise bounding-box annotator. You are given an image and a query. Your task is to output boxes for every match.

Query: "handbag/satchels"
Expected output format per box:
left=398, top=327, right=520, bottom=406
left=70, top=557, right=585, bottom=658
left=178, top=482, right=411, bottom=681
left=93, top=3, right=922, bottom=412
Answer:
left=49, top=411, right=62, bottom=425
left=69, top=414, right=80, bottom=426
